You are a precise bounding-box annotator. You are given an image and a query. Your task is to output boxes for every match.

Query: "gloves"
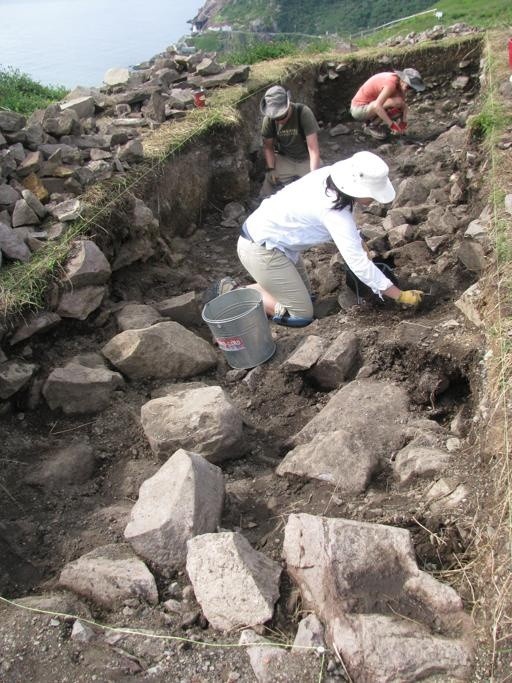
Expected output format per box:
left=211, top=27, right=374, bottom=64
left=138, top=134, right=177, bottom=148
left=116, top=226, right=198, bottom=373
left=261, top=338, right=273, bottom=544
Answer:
left=398, top=289, right=426, bottom=310
left=389, top=121, right=408, bottom=136
left=265, top=168, right=279, bottom=186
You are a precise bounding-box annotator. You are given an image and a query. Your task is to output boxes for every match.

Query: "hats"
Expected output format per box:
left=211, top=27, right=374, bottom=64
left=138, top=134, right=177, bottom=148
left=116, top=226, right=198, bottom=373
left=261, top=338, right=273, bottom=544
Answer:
left=394, top=68, right=426, bottom=92
left=259, top=86, right=291, bottom=119
left=330, top=150, right=396, bottom=204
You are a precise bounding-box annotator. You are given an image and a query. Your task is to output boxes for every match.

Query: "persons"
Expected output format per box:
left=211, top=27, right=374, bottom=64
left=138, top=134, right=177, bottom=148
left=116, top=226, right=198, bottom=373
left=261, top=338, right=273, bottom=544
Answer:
left=349, top=67, right=426, bottom=140
left=216, top=149, right=423, bottom=329
left=257, top=85, right=321, bottom=199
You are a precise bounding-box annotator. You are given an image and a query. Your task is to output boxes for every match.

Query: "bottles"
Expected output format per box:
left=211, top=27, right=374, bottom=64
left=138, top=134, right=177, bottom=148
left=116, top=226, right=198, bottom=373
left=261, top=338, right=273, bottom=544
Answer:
left=200, top=85, right=204, bottom=91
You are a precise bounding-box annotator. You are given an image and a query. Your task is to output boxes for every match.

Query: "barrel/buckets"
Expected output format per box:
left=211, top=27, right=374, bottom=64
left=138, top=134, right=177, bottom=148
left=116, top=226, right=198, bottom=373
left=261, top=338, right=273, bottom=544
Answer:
left=191, top=89, right=206, bottom=108
left=507, top=37, right=512, bottom=68
left=200, top=288, right=278, bottom=371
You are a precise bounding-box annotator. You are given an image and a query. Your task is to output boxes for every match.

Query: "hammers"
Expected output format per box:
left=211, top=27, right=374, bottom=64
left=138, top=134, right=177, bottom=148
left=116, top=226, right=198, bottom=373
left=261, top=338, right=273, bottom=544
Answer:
left=268, top=174, right=280, bottom=189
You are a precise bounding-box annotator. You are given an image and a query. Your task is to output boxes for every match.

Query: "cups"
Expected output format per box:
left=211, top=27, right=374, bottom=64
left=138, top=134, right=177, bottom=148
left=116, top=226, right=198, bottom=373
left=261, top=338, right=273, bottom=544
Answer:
left=194, top=89, right=205, bottom=107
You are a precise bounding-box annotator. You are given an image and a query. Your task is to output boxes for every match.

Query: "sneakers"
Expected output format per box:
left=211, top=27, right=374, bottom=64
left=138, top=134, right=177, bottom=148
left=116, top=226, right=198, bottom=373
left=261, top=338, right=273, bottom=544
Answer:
left=364, top=120, right=387, bottom=140
